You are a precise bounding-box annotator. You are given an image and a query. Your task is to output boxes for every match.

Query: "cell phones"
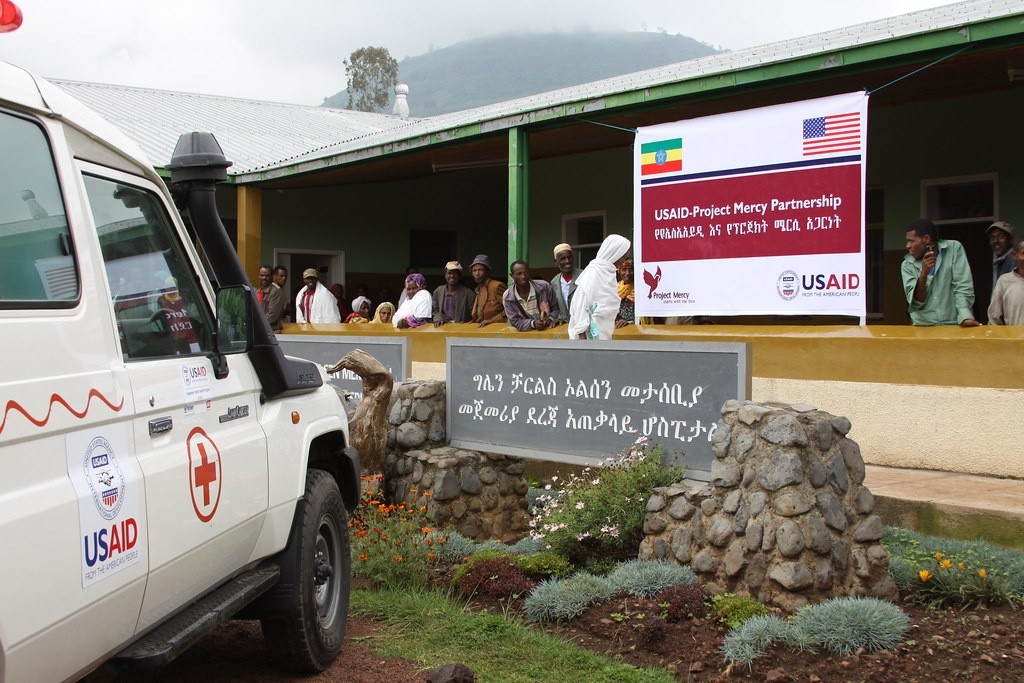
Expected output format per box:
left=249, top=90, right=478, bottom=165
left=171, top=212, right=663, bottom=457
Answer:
left=926, top=245, right=935, bottom=253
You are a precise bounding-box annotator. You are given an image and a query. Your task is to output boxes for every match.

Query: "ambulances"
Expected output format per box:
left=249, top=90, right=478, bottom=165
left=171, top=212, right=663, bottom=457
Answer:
left=0, top=53, right=362, bottom=683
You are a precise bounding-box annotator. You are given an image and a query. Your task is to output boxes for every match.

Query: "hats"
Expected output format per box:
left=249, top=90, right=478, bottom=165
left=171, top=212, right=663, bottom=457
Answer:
left=618, top=257, right=634, bottom=268
left=446, top=261, right=463, bottom=271
left=553, top=243, right=572, bottom=259
left=468, top=255, right=493, bottom=271
left=985, top=221, right=1015, bottom=236
left=303, top=268, right=319, bottom=279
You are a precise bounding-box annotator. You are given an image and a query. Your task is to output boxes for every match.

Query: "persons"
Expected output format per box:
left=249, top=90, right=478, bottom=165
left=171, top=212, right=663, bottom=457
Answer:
left=368, top=302, right=396, bottom=324
left=615, top=257, right=634, bottom=329
left=549, top=243, right=584, bottom=329
left=393, top=275, right=432, bottom=328
left=252, top=265, right=289, bottom=333
left=468, top=254, right=508, bottom=328
left=986, top=221, right=1024, bottom=327
left=900, top=218, right=983, bottom=328
left=330, top=283, right=349, bottom=322
left=398, top=265, right=420, bottom=310
left=343, top=295, right=372, bottom=322
left=273, top=265, right=295, bottom=323
left=503, top=262, right=559, bottom=332
left=296, top=268, right=341, bottom=324
left=568, top=233, right=632, bottom=341
left=432, top=261, right=476, bottom=328
